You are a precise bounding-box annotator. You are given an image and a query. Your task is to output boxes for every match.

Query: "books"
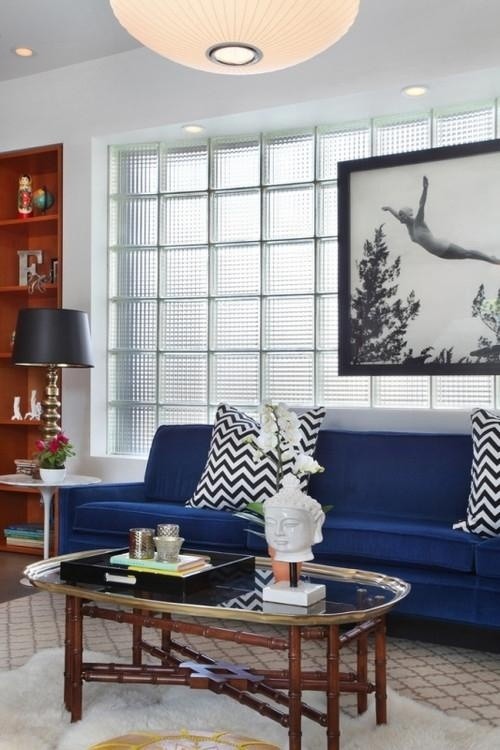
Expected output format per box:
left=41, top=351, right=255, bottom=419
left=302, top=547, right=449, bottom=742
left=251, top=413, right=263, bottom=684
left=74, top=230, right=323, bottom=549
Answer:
left=3, top=523, right=44, bottom=549
left=128, top=564, right=214, bottom=579
left=110, top=550, right=208, bottom=572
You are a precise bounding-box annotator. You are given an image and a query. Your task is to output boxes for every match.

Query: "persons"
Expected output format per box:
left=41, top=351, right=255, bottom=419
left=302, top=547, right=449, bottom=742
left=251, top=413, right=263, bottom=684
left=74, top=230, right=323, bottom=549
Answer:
left=380, top=174, right=499, bottom=268
left=263, top=485, right=326, bottom=563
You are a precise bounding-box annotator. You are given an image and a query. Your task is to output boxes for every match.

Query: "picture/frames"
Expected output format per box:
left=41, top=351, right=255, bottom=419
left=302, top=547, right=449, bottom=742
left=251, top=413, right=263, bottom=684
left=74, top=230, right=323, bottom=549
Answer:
left=50, top=257, right=58, bottom=284
left=339, top=139, right=499, bottom=375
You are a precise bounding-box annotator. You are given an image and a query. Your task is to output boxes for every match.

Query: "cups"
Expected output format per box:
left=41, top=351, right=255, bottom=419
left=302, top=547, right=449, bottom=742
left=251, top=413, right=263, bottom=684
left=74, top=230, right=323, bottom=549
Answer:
left=153, top=537, right=185, bottom=562
left=154, top=523, right=180, bottom=537
left=128, top=527, right=155, bottom=558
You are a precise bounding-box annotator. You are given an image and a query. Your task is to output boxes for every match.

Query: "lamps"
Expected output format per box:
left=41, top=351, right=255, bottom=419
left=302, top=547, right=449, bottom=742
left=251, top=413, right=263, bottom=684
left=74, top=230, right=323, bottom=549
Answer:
left=107, top=0, right=361, bottom=76
left=10, top=307, right=93, bottom=478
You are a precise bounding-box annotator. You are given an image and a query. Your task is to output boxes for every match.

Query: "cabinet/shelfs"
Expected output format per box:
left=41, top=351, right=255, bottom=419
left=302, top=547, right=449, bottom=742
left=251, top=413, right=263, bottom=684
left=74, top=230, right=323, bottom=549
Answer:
left=1, top=141, right=65, bottom=557
left=2, top=472, right=101, bottom=562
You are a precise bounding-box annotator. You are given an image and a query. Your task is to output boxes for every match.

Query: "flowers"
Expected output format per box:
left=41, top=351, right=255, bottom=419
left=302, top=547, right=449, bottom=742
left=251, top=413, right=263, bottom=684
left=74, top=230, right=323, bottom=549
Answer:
left=247, top=401, right=319, bottom=518
left=32, top=434, right=73, bottom=468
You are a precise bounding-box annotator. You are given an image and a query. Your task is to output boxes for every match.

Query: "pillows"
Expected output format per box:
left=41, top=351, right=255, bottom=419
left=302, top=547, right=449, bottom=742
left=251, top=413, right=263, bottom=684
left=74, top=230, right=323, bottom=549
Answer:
left=182, top=402, right=328, bottom=514
left=453, top=408, right=500, bottom=539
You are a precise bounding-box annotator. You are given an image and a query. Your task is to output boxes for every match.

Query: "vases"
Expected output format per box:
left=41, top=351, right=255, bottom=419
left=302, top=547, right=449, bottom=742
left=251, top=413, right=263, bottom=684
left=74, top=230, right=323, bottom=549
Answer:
left=39, top=468, right=66, bottom=484
left=269, top=541, right=305, bottom=584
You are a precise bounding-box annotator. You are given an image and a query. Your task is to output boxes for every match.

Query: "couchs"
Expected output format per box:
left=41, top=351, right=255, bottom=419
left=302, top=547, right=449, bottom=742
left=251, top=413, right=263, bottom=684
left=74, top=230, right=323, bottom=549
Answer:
left=49, top=407, right=500, bottom=643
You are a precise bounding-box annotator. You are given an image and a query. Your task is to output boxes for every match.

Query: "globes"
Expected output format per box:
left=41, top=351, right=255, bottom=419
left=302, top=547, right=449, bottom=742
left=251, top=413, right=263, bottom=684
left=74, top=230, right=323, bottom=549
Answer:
left=31, top=185, right=55, bottom=216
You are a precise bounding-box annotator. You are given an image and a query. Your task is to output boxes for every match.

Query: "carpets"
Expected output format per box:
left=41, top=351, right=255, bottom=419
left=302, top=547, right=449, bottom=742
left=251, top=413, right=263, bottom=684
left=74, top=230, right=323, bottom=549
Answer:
left=1, top=647, right=500, bottom=749
left=1, top=590, right=500, bottom=748
